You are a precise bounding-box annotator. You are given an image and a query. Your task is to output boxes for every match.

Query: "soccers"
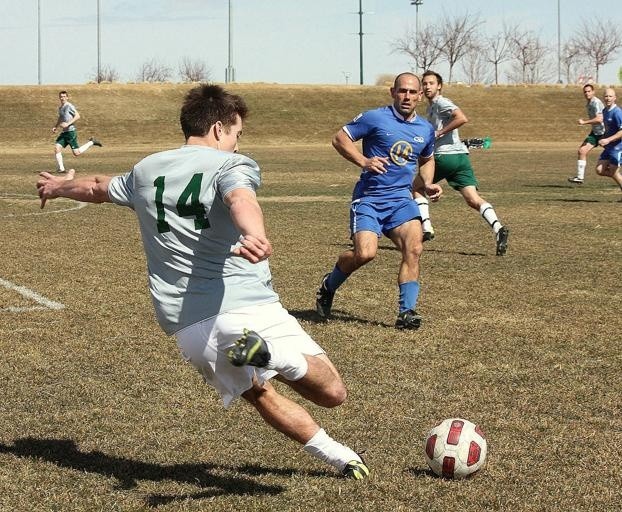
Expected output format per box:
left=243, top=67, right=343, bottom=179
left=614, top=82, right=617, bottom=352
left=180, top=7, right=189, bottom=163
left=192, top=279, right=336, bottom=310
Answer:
left=424, top=418, right=487, bottom=480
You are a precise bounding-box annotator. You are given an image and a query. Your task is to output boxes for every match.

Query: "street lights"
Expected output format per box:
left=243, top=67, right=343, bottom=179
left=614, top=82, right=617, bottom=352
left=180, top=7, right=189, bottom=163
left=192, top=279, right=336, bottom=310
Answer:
left=411, top=0, right=423, bottom=70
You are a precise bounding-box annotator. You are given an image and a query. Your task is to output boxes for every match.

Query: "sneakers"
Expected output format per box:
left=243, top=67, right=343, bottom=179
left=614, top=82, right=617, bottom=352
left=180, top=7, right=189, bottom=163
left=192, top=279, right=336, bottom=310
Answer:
left=341, top=452, right=371, bottom=481
left=422, top=225, right=435, bottom=244
left=316, top=272, right=336, bottom=319
left=495, top=225, right=510, bottom=257
left=394, top=308, right=423, bottom=330
left=567, top=176, right=586, bottom=185
left=54, top=169, right=66, bottom=173
left=226, top=327, right=271, bottom=368
left=89, top=137, right=102, bottom=148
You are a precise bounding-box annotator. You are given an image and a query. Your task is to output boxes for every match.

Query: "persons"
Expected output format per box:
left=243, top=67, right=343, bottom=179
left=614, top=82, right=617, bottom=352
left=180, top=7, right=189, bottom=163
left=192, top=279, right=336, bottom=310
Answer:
left=315, top=71, right=443, bottom=329
left=567, top=83, right=605, bottom=185
left=37, top=81, right=370, bottom=481
left=411, top=70, right=509, bottom=258
left=596, top=88, right=622, bottom=191
left=49, top=91, right=103, bottom=174
